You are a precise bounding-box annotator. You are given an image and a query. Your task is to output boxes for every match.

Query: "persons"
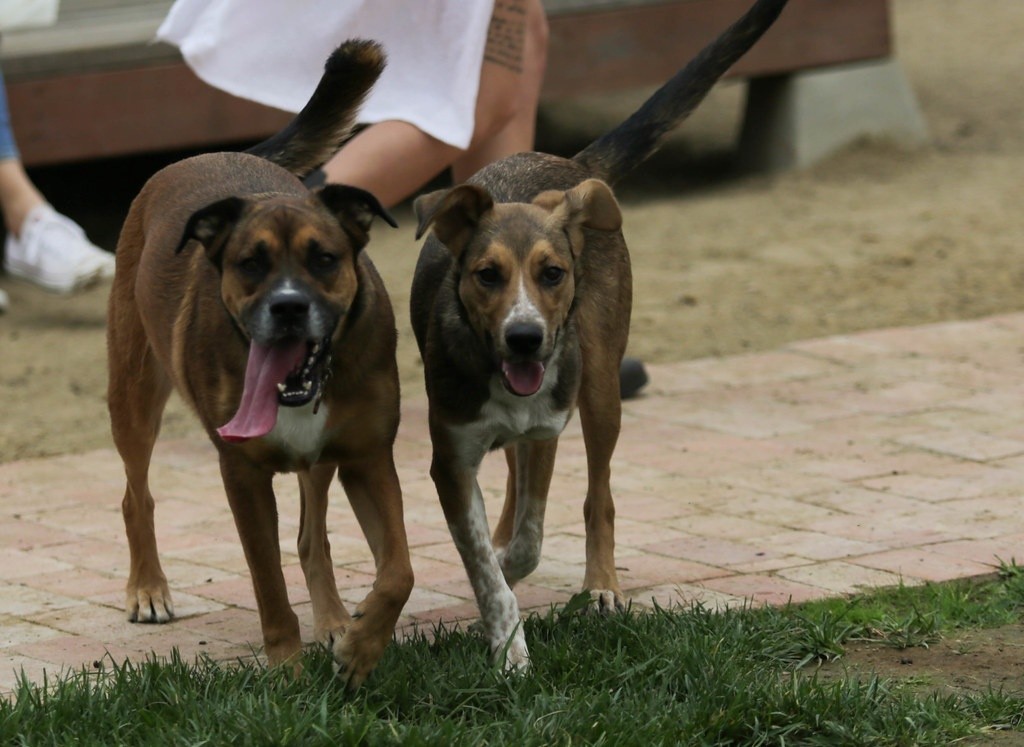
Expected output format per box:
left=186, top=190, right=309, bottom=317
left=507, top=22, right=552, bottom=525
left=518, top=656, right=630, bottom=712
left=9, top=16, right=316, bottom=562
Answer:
left=0, top=72, right=116, bottom=314
left=157, top=0, right=647, bottom=400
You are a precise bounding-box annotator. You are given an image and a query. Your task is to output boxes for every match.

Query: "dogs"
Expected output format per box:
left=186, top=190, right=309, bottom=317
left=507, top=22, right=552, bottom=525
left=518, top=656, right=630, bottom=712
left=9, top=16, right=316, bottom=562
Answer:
left=105, top=39, right=414, bottom=695
left=408, top=1, right=788, bottom=682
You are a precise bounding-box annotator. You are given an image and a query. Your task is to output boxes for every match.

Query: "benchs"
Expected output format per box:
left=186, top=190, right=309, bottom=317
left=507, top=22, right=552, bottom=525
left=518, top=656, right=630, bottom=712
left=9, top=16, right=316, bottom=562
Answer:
left=0, top=0, right=932, bottom=177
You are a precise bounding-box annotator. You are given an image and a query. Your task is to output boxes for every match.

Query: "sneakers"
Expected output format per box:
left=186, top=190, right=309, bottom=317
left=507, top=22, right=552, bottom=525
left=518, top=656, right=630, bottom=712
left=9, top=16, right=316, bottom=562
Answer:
left=3, top=205, right=116, bottom=293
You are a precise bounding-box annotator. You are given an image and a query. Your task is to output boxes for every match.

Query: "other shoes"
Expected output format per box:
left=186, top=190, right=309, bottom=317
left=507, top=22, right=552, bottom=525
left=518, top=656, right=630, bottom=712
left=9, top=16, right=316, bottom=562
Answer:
left=618, top=358, right=646, bottom=397
